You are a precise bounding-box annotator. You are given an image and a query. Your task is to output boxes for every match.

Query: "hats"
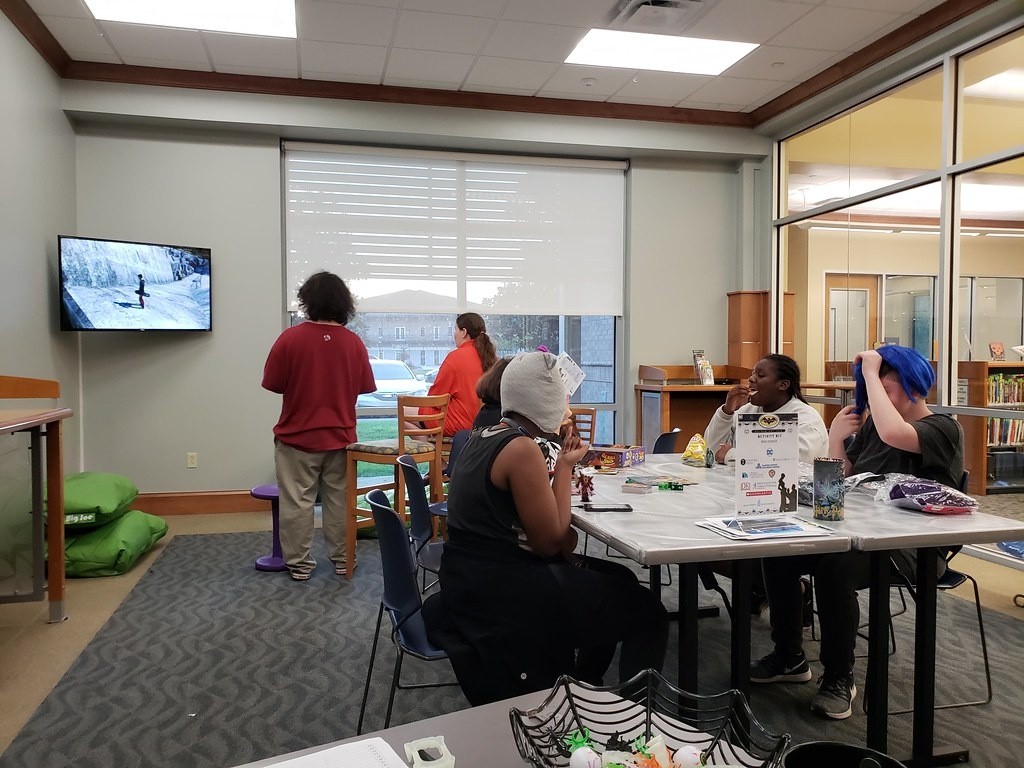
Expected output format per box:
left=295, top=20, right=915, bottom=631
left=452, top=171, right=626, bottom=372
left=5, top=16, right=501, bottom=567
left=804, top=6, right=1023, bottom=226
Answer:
left=501, top=352, right=568, bottom=434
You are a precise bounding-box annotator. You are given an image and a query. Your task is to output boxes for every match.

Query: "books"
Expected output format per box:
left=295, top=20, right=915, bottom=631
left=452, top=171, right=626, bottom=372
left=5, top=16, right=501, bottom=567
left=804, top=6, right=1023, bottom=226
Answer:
left=987, top=374, right=1024, bottom=446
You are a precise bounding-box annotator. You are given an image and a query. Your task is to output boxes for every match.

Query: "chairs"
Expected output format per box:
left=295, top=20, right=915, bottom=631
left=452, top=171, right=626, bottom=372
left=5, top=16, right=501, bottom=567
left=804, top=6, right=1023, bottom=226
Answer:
left=796, top=427, right=869, bottom=643
left=569, top=405, right=597, bottom=448
left=354, top=486, right=465, bottom=736
left=578, top=420, right=685, bottom=586
left=809, top=460, right=996, bottom=722
left=389, top=451, right=450, bottom=595
left=427, top=419, right=476, bottom=543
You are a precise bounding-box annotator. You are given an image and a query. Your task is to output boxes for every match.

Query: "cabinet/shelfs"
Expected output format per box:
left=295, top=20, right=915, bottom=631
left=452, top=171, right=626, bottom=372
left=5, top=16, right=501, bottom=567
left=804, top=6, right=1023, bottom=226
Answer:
left=957, top=358, right=1024, bottom=497
left=628, top=380, right=752, bottom=456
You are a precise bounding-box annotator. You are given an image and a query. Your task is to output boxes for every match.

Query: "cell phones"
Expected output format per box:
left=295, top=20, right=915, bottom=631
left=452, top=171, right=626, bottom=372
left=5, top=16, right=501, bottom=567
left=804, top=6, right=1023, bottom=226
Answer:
left=584, top=503, right=633, bottom=512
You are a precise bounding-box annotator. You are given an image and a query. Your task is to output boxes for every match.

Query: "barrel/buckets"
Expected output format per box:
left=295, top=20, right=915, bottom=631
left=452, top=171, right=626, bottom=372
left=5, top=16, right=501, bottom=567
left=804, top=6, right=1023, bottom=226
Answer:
left=779, top=741, right=907, bottom=768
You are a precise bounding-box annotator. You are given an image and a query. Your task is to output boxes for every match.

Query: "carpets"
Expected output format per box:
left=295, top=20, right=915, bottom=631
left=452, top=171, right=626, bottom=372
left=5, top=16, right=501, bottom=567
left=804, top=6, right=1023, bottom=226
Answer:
left=0, top=527, right=1024, bottom=768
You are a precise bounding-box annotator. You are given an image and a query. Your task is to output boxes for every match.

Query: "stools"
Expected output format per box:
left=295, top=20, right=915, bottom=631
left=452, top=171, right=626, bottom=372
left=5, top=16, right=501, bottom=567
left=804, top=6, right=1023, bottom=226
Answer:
left=248, top=481, right=289, bottom=572
left=341, top=392, right=455, bottom=582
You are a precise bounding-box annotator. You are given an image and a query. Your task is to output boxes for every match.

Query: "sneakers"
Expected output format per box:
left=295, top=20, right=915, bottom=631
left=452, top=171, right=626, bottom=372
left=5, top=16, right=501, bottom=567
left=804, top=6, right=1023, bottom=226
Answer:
left=750, top=650, right=812, bottom=683
left=811, top=673, right=857, bottom=720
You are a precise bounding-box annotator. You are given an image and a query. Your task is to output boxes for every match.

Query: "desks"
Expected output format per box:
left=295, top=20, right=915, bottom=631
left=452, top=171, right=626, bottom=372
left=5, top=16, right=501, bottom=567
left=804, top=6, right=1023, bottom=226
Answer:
left=213, top=449, right=1024, bottom=768
left=0, top=401, right=76, bottom=627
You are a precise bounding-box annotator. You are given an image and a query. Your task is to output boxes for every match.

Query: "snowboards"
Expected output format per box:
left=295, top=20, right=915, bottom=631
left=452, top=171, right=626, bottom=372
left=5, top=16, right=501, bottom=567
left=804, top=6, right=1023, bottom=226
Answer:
left=135, top=290, right=151, bottom=297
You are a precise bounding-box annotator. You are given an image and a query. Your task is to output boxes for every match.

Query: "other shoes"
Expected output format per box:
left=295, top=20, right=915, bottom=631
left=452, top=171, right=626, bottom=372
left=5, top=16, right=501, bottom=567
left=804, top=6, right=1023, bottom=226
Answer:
left=289, top=569, right=310, bottom=580
left=335, top=552, right=358, bottom=574
left=800, top=578, right=813, bottom=627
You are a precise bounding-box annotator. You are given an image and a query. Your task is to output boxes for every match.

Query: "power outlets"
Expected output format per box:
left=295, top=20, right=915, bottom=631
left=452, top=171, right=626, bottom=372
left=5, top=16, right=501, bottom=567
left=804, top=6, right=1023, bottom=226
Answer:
left=186, top=452, right=198, bottom=467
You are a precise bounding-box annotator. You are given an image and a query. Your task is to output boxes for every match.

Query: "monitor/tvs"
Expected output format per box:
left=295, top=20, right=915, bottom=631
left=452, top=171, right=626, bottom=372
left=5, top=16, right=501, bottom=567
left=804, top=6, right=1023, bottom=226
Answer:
left=57, top=234, right=212, bottom=332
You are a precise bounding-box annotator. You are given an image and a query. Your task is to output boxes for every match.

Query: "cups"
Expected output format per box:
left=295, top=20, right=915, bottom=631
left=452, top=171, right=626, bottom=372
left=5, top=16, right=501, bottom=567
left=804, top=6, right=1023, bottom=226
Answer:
left=813, top=457, right=846, bottom=522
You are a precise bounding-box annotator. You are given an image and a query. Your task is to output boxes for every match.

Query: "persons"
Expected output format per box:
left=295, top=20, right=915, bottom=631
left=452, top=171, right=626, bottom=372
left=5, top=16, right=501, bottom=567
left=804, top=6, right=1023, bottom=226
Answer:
left=401, top=313, right=499, bottom=484
left=747, top=345, right=965, bottom=720
left=261, top=271, right=378, bottom=580
left=702, top=355, right=830, bottom=632
left=439, top=352, right=671, bottom=708
left=136, top=274, right=146, bottom=308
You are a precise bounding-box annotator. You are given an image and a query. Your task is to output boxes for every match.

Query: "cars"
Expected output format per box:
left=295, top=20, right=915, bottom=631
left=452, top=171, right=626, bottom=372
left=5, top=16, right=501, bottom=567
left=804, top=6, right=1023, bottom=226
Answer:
left=356, top=355, right=439, bottom=408
